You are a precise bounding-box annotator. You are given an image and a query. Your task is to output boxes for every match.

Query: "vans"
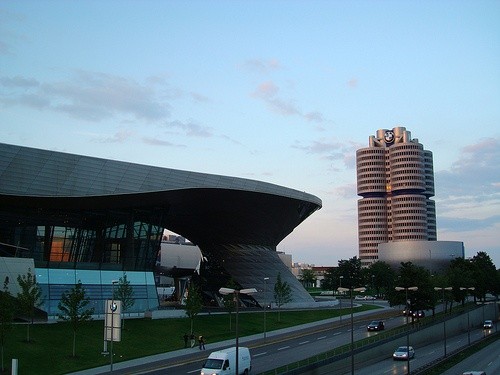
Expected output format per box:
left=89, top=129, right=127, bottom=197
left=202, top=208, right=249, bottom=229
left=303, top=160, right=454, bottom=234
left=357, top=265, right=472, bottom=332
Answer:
left=201, top=347, right=251, bottom=374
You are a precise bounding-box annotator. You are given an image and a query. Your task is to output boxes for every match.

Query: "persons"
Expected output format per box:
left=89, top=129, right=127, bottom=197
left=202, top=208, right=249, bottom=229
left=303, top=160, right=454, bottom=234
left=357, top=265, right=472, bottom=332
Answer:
left=190, top=332, right=195, bottom=348
left=198, top=335, right=205, bottom=350
left=183, top=332, right=189, bottom=348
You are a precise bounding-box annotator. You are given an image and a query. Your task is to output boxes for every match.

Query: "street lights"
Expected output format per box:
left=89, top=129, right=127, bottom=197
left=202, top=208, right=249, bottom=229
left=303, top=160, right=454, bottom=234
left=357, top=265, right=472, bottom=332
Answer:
left=338, top=287, right=366, bottom=375
left=110, top=280, right=119, bottom=373
left=459, top=286, right=475, bottom=344
left=396, top=285, right=418, bottom=375
left=434, top=286, right=453, bottom=361
left=219, top=288, right=258, bottom=375
left=264, top=277, right=270, bottom=343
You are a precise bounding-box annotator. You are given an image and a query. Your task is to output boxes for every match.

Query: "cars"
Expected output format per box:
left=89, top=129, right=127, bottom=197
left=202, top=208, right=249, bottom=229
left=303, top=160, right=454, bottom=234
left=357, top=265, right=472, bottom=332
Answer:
left=412, top=310, right=425, bottom=317
left=393, top=346, right=415, bottom=360
left=463, top=371, right=486, bottom=375
left=367, top=321, right=384, bottom=331
left=484, top=320, right=493, bottom=328
